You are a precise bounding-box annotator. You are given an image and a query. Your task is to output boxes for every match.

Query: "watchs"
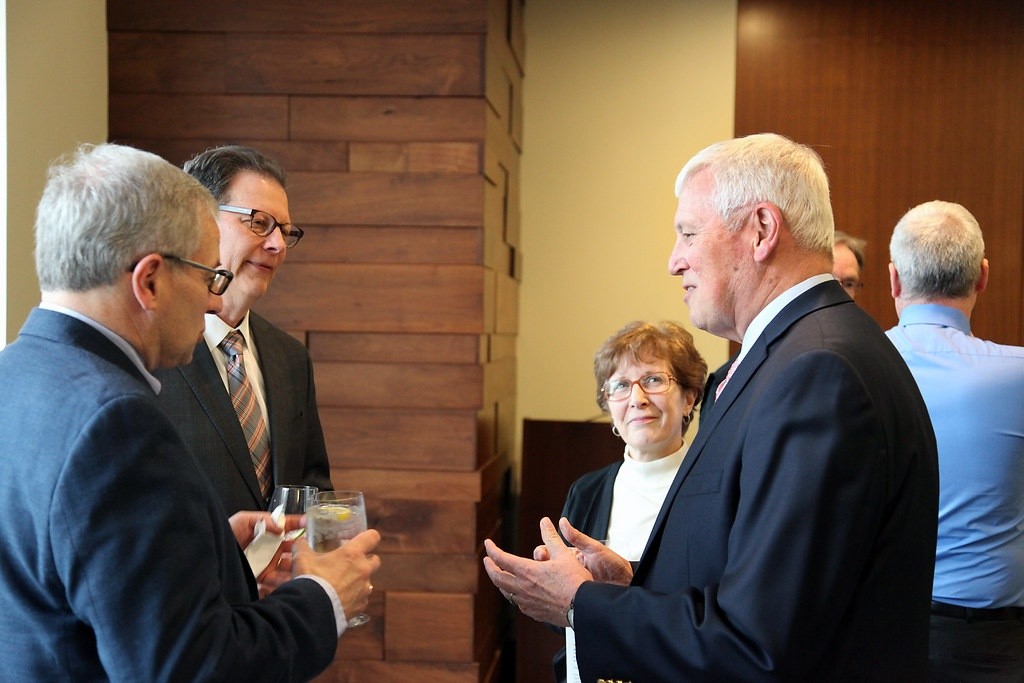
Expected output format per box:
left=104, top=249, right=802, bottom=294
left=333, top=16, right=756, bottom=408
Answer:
left=566, top=595, right=574, bottom=630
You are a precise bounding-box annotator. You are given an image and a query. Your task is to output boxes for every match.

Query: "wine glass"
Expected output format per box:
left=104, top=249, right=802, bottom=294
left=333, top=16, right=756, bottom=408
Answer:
left=306, top=490, right=371, bottom=628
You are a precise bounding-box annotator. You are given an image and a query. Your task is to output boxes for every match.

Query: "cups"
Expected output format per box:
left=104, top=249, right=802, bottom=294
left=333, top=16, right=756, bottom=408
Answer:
left=267, top=485, right=319, bottom=540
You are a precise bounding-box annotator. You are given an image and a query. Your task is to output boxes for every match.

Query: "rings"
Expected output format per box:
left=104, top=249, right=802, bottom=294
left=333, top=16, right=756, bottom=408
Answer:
left=276, top=556, right=283, bottom=567
left=508, top=592, right=515, bottom=605
left=368, top=585, right=373, bottom=593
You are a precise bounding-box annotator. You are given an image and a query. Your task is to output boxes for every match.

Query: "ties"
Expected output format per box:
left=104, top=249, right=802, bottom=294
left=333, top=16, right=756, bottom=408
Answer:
left=218, top=331, right=274, bottom=505
left=715, top=359, right=738, bottom=403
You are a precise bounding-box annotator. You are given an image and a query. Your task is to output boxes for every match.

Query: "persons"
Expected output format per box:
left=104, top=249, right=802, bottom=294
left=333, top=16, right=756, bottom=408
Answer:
left=883, top=200, right=1024, bottom=682
left=1, top=142, right=382, bottom=683
left=482, top=131, right=939, bottom=683
left=183, top=145, right=338, bottom=518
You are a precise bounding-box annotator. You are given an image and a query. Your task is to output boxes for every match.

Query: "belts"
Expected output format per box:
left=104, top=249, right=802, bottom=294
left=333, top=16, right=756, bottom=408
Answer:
left=930, top=600, right=1024, bottom=621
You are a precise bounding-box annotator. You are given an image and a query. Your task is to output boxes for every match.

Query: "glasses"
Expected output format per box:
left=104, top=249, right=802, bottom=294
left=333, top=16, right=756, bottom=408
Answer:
left=218, top=204, right=304, bottom=250
left=129, top=251, right=233, bottom=296
left=601, top=372, right=682, bottom=401
left=839, top=280, right=865, bottom=296
